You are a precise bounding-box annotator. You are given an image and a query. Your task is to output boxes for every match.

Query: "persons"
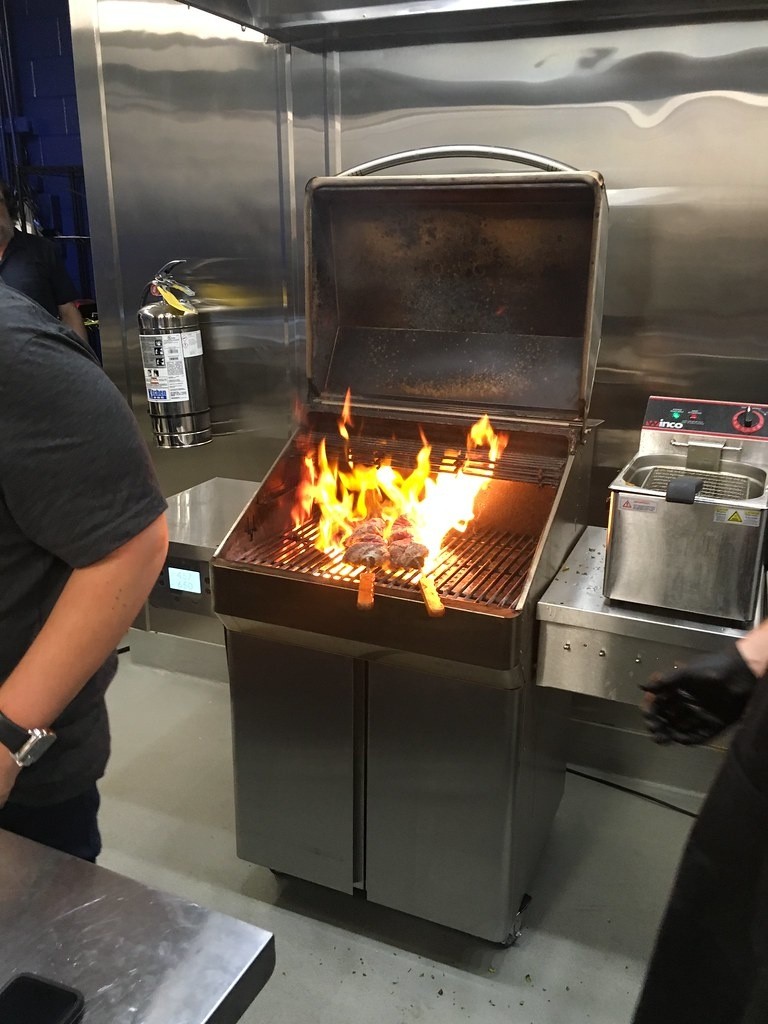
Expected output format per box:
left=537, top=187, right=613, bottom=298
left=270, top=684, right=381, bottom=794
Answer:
left=629, top=620, right=767, bottom=1024
left=0, top=284, right=169, bottom=865
left=0, top=181, right=88, bottom=342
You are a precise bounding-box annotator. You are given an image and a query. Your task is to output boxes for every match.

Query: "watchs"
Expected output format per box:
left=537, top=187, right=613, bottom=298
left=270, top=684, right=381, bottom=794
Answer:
left=0, top=708, right=57, bottom=767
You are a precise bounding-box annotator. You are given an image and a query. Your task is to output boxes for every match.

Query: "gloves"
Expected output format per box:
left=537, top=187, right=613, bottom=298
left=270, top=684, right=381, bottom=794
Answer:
left=639, top=641, right=758, bottom=746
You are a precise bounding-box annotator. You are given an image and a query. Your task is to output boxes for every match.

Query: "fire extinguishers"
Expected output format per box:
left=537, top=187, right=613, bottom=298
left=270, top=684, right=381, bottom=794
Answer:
left=136, top=259, right=213, bottom=449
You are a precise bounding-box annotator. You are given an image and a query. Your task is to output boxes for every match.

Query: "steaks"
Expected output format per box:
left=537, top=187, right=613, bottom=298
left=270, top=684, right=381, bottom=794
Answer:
left=342, top=513, right=429, bottom=569
